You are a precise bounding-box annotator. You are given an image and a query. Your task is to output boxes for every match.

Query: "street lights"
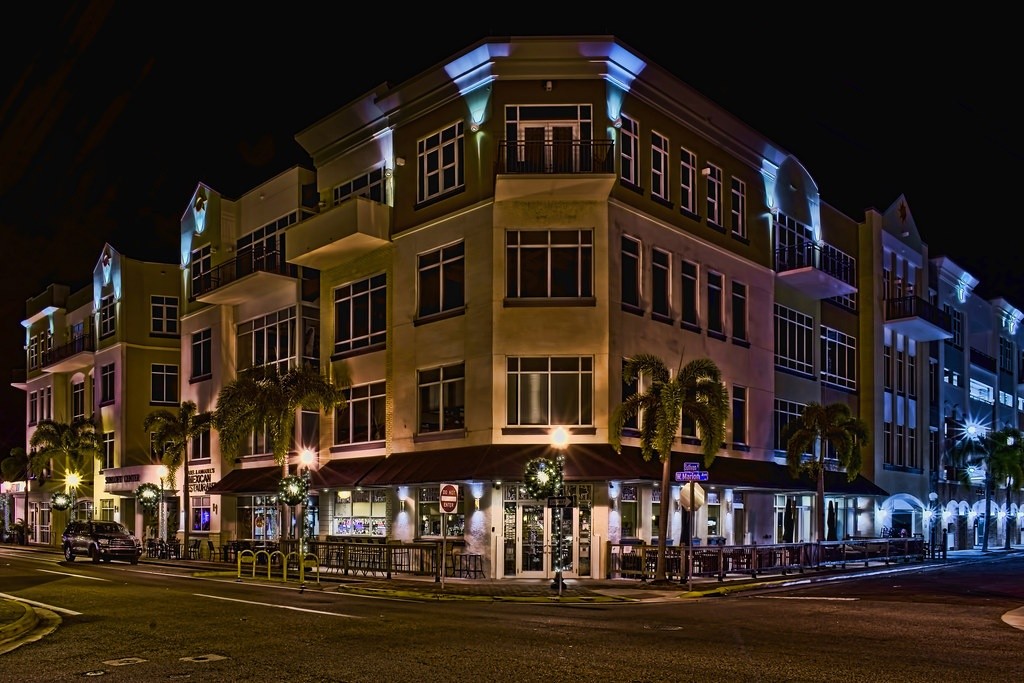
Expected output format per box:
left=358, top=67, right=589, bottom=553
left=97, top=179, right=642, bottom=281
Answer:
left=298, top=450, right=315, bottom=572
left=1, top=481, right=13, bottom=543
left=156, top=465, right=170, bottom=559
left=548, top=426, right=571, bottom=589
left=66, top=472, right=80, bottom=520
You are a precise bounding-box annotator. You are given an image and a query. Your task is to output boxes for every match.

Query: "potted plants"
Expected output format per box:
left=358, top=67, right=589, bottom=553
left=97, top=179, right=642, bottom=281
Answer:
left=13, top=518, right=31, bottom=545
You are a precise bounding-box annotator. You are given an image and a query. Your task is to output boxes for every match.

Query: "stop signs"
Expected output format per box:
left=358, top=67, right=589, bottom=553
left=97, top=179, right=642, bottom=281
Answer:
left=440, top=485, right=458, bottom=512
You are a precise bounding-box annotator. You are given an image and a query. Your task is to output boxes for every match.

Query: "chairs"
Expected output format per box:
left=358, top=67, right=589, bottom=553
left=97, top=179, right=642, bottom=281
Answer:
left=144, top=537, right=201, bottom=560
left=644, top=545, right=824, bottom=576
left=208, top=540, right=279, bottom=563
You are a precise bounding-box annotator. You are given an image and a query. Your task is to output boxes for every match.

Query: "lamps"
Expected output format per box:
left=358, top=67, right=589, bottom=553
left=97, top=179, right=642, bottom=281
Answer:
left=816, top=240, right=824, bottom=247
left=612, top=118, right=622, bottom=128
left=770, top=207, right=779, bottom=214
left=385, top=169, right=394, bottom=178
left=471, top=124, right=480, bottom=133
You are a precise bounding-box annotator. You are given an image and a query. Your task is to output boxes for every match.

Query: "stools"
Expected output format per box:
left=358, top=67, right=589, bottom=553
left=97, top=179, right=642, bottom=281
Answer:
left=317, top=546, right=486, bottom=578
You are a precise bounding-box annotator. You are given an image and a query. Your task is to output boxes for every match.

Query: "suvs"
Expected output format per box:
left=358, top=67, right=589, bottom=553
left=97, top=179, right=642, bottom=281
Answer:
left=62, top=518, right=142, bottom=565
